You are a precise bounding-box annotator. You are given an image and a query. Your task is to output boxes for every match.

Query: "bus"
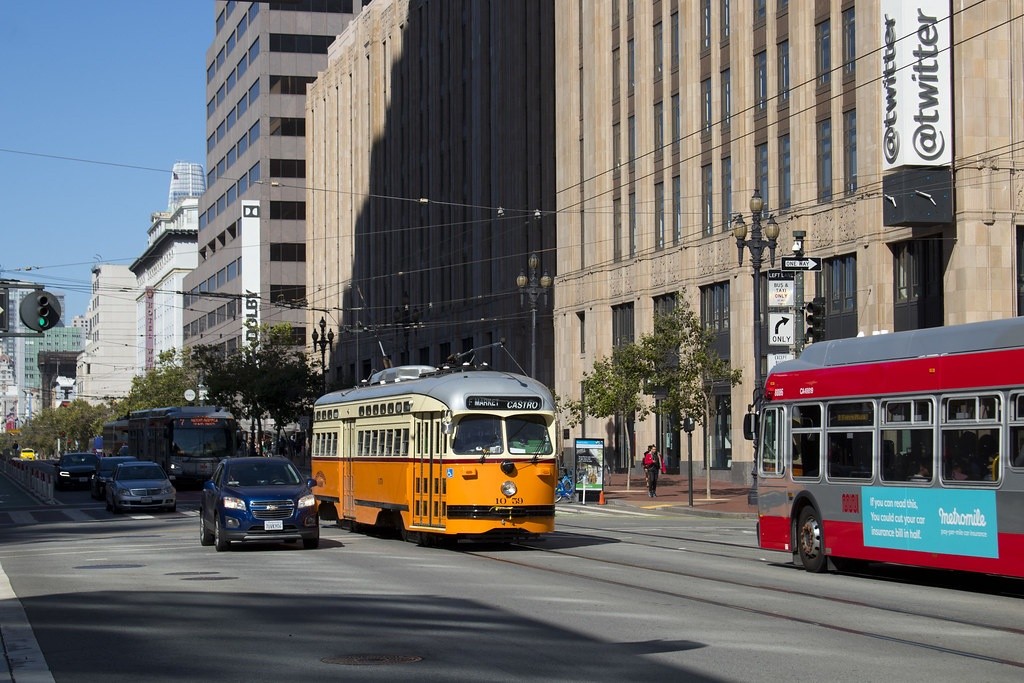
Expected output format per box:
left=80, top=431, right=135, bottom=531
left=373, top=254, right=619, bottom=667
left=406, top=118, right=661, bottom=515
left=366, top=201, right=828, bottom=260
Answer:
left=309, top=328, right=557, bottom=549
left=742, top=316, right=1023, bottom=579
left=103, top=413, right=130, bottom=457
left=128, top=405, right=243, bottom=488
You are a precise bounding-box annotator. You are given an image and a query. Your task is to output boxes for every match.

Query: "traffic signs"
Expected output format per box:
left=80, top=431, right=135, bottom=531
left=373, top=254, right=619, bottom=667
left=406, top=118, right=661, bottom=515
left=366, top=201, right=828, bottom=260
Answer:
left=780, top=255, right=823, bottom=273
left=767, top=311, right=797, bottom=346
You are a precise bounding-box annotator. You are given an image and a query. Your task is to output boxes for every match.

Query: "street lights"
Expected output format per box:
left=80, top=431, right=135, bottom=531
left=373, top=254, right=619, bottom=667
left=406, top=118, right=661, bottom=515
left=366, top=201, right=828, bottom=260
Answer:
left=515, top=250, right=551, bottom=380
left=312, top=317, right=335, bottom=397
left=735, top=186, right=780, bottom=504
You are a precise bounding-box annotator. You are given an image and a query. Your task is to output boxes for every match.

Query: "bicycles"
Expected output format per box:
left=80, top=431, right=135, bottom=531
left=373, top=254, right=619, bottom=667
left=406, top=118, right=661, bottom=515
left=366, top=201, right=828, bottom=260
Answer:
left=555, top=465, right=574, bottom=503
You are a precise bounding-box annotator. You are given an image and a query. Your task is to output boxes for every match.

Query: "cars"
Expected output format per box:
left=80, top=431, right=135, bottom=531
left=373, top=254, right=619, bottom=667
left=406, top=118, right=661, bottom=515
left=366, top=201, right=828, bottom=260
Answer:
left=53, top=452, right=101, bottom=492
left=19, top=448, right=35, bottom=460
left=90, top=456, right=137, bottom=502
left=198, top=455, right=319, bottom=553
left=106, top=461, right=177, bottom=513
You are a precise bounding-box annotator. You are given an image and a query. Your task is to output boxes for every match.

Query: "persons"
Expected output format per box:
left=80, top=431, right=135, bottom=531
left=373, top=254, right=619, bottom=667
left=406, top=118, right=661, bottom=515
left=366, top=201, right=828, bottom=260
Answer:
left=810, top=432, right=1024, bottom=481
left=278, top=437, right=299, bottom=456
left=264, top=438, right=272, bottom=451
left=641, top=445, right=666, bottom=497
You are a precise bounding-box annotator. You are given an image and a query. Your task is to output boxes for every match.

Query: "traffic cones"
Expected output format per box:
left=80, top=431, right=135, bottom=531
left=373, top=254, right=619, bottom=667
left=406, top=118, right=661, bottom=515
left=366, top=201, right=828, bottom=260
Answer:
left=597, top=490, right=606, bottom=505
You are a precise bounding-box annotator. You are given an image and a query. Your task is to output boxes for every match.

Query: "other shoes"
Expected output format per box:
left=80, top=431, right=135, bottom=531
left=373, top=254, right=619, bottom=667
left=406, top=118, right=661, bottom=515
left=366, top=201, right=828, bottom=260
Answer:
left=649, top=492, right=653, bottom=497
left=654, top=493, right=656, bottom=497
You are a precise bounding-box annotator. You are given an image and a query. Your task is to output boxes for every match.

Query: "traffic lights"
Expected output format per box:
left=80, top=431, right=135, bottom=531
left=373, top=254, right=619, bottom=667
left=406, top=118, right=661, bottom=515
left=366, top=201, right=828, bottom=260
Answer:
left=806, top=301, right=825, bottom=342
left=20, top=291, right=61, bottom=331
